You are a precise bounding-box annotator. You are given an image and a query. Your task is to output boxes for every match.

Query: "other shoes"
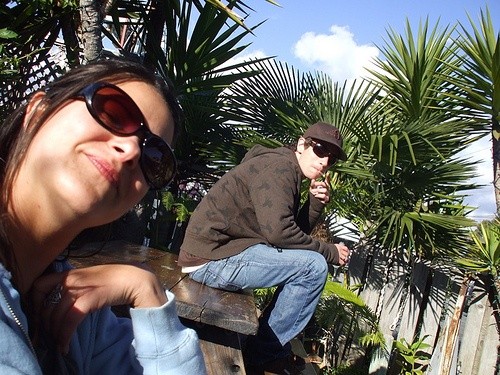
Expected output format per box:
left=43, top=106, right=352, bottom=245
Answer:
left=256, top=352, right=306, bottom=375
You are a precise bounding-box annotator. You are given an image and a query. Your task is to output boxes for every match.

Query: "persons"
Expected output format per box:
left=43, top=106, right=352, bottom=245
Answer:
left=148, top=179, right=186, bottom=248
left=0, top=57, right=207, bottom=375
left=179, top=179, right=201, bottom=201
left=178, top=122, right=352, bottom=375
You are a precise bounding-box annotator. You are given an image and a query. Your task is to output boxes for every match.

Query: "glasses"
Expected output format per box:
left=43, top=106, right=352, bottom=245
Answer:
left=310, top=139, right=337, bottom=167
left=75, top=81, right=175, bottom=189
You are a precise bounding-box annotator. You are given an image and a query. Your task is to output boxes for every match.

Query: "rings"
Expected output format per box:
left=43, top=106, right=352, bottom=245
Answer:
left=47, top=286, right=63, bottom=304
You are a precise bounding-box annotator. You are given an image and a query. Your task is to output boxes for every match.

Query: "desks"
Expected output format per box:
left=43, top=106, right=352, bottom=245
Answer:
left=63, top=237, right=258, bottom=375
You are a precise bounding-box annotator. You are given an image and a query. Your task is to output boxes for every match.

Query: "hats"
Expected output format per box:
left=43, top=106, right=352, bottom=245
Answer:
left=303, top=122, right=349, bottom=163
